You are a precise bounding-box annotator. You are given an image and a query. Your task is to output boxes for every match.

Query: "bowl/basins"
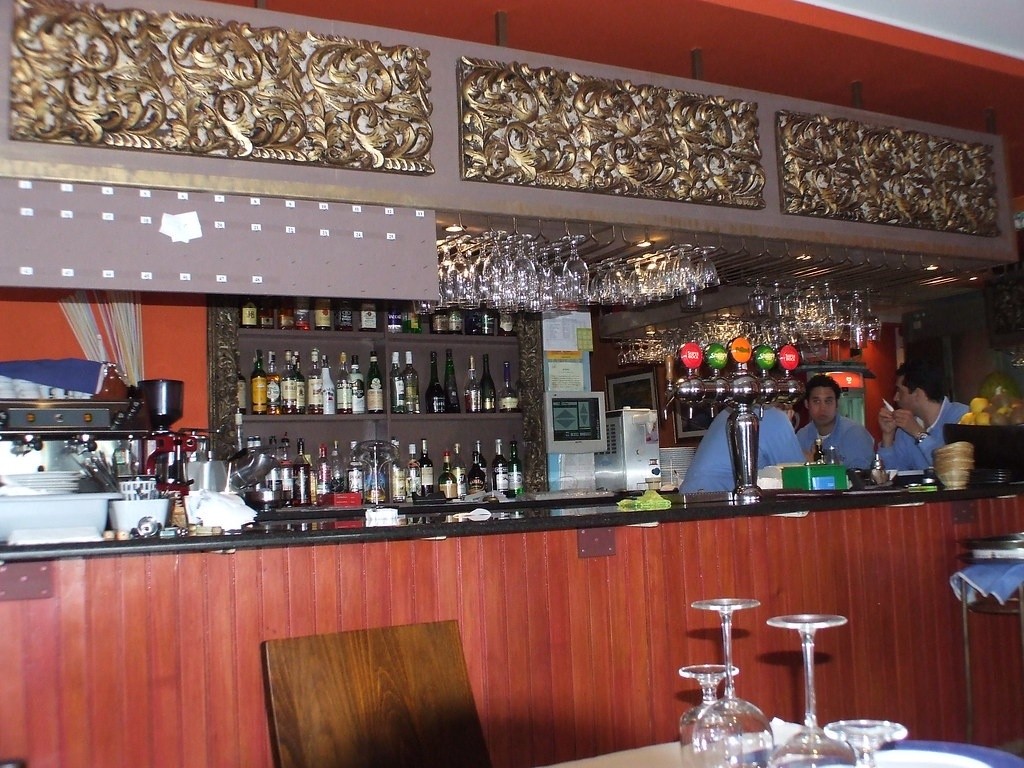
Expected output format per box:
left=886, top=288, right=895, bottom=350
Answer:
left=931, top=442, right=975, bottom=490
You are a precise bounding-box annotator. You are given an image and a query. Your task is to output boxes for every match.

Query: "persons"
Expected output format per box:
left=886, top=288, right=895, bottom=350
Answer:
left=679, top=404, right=806, bottom=493
left=877, top=357, right=969, bottom=470
left=796, top=375, right=876, bottom=469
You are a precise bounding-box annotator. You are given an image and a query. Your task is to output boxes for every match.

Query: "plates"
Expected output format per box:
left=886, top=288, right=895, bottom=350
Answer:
left=0, top=471, right=80, bottom=494
left=659, top=447, right=696, bottom=486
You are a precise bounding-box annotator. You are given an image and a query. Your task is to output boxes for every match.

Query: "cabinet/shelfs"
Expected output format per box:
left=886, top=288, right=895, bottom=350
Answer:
left=205, top=310, right=547, bottom=523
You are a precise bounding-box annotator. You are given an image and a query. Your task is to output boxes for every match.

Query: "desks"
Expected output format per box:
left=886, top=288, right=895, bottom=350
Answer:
left=545, top=738, right=1024, bottom=768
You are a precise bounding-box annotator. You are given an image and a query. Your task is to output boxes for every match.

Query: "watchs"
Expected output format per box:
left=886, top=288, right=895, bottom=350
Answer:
left=915, top=432, right=930, bottom=443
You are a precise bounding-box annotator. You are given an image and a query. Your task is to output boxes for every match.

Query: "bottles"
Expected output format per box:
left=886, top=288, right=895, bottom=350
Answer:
left=870, top=454, right=886, bottom=484
left=235, top=348, right=384, bottom=415
left=238, top=432, right=384, bottom=509
left=390, top=349, right=520, bottom=415
left=241, top=295, right=376, bottom=330
left=386, top=297, right=516, bottom=335
left=813, top=439, right=825, bottom=461
left=390, top=437, right=521, bottom=502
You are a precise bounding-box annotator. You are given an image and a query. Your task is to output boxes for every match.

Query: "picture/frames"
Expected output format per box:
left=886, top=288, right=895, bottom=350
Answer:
left=605, top=367, right=663, bottom=431
left=672, top=398, right=725, bottom=443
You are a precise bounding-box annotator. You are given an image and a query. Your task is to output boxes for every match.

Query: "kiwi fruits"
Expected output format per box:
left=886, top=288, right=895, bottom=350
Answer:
left=983, top=393, right=1024, bottom=425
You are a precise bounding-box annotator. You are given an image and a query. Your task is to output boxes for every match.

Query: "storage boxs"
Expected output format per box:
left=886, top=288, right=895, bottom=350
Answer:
left=781, top=465, right=847, bottom=491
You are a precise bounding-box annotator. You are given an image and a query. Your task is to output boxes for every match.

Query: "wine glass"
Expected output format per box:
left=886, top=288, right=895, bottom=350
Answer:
left=679, top=597, right=908, bottom=767
left=412, top=228, right=881, bottom=363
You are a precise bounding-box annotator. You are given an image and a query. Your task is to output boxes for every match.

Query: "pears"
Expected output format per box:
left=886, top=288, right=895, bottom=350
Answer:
left=956, top=397, right=995, bottom=425
